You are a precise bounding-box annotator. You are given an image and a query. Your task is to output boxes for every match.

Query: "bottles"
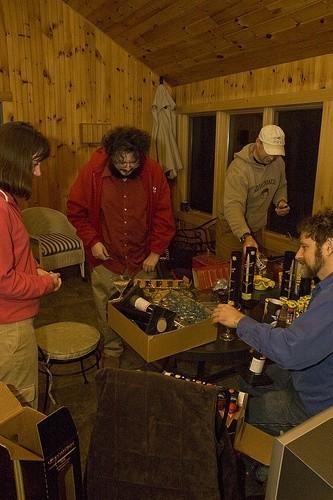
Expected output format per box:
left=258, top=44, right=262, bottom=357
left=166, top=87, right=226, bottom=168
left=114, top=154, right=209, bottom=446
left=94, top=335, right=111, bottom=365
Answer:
left=247, top=348, right=266, bottom=377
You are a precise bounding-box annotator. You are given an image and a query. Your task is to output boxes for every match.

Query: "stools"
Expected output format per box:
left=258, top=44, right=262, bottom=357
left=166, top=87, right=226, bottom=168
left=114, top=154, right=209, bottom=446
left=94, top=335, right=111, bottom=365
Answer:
left=35, top=321, right=102, bottom=413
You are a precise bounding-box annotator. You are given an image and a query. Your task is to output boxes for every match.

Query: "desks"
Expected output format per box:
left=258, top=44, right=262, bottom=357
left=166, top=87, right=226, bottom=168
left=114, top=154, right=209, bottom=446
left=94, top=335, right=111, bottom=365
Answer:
left=105, top=274, right=301, bottom=383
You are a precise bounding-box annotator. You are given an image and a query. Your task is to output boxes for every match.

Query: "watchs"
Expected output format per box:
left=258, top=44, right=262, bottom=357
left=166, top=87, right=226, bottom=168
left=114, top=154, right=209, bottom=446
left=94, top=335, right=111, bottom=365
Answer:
left=240, top=233, right=251, bottom=243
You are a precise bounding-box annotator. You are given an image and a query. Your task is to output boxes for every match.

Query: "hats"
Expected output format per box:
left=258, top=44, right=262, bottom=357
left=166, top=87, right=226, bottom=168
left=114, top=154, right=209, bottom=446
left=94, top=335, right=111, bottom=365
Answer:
left=259, top=125, right=286, bottom=156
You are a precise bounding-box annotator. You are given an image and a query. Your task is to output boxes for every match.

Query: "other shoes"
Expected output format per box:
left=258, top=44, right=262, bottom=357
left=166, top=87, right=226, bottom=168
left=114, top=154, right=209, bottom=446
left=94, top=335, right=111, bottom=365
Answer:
left=100, top=356, right=121, bottom=369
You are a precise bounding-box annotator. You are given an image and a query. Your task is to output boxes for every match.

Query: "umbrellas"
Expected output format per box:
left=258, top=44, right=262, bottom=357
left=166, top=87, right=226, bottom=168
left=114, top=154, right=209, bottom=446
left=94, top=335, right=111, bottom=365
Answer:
left=147, top=79, right=183, bottom=174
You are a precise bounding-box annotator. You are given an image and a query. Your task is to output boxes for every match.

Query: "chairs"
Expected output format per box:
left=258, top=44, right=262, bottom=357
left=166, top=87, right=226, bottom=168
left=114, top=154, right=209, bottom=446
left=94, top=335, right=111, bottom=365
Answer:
left=82, top=367, right=247, bottom=500
left=157, top=217, right=218, bottom=280
left=23, top=207, right=84, bottom=280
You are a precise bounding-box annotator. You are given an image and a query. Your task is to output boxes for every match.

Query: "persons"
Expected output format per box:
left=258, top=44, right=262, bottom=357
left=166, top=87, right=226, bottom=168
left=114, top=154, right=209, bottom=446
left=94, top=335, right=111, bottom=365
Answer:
left=66, top=127, right=177, bottom=369
left=0, top=122, right=62, bottom=410
left=216, top=124, right=290, bottom=266
left=212, top=205, right=333, bottom=480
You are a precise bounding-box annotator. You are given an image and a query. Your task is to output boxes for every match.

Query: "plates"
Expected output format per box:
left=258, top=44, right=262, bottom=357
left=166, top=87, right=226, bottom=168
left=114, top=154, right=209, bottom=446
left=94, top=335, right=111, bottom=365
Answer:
left=253, top=275, right=275, bottom=290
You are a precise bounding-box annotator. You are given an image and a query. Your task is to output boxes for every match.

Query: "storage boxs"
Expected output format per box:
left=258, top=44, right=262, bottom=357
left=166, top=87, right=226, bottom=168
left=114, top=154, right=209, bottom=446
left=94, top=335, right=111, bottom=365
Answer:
left=108, top=289, right=217, bottom=362
left=0, top=381, right=83, bottom=500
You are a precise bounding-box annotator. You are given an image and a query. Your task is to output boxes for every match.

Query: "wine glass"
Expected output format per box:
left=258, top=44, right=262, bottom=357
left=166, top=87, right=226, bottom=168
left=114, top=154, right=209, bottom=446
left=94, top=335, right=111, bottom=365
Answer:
left=216, top=301, right=242, bottom=342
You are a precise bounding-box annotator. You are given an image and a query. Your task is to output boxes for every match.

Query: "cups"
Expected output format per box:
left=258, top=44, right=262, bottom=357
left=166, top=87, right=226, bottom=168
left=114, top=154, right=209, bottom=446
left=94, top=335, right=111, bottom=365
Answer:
left=263, top=298, right=285, bottom=328
